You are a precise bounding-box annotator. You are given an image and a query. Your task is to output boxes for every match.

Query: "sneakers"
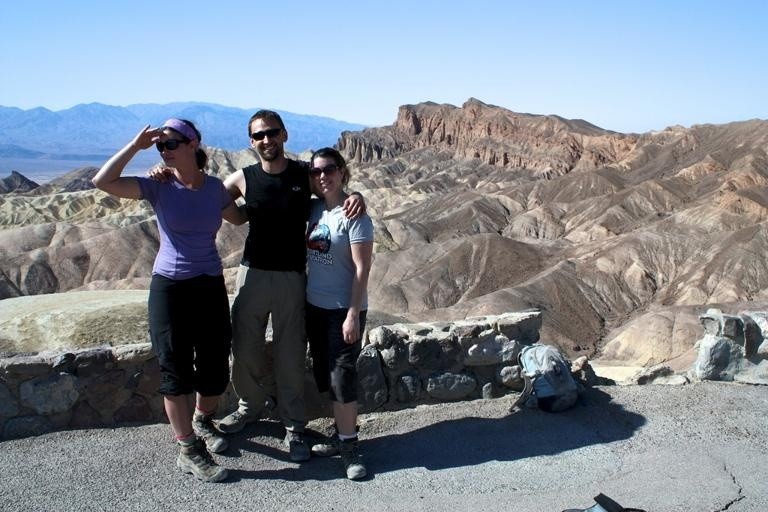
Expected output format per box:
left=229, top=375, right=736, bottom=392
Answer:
left=191, top=414, right=230, bottom=453
left=339, top=440, right=368, bottom=481
left=218, top=409, right=257, bottom=434
left=176, top=435, right=229, bottom=483
left=312, top=432, right=340, bottom=459
left=284, top=427, right=311, bottom=461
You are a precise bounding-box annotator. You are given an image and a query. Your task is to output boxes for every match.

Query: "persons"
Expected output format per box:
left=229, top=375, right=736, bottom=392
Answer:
left=303, top=147, right=373, bottom=479
left=148, top=109, right=366, bottom=462
left=92, top=118, right=249, bottom=483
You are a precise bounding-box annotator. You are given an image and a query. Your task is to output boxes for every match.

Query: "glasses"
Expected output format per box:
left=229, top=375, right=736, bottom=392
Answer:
left=250, top=126, right=285, bottom=141
left=155, top=138, right=186, bottom=152
left=309, top=164, right=345, bottom=178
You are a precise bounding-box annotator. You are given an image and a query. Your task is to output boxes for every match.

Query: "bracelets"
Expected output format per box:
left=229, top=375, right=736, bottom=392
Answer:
left=350, top=192, right=363, bottom=200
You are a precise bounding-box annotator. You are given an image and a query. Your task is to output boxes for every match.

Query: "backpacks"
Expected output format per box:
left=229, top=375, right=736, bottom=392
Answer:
left=507, top=342, right=586, bottom=415
